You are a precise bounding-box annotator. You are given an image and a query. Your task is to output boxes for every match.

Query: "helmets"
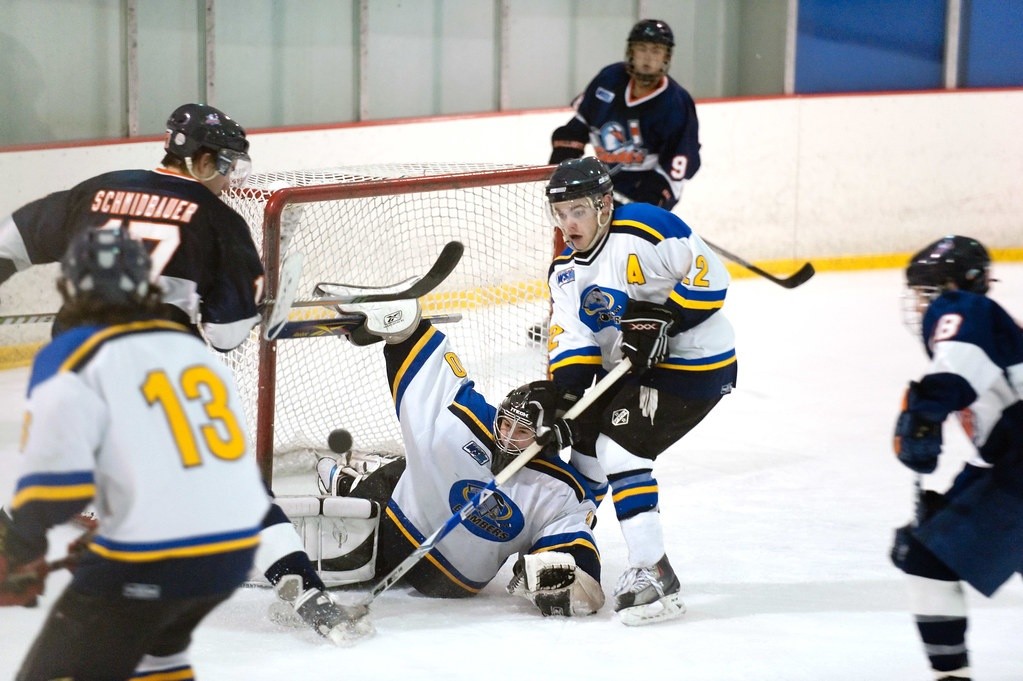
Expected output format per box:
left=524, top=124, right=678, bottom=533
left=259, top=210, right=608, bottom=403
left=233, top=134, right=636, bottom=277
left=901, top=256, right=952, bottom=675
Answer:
left=60, top=226, right=152, bottom=307
left=900, top=235, right=991, bottom=344
left=626, top=18, right=675, bottom=87
left=544, top=156, right=614, bottom=238
left=164, top=103, right=253, bottom=164
left=493, top=382, right=557, bottom=459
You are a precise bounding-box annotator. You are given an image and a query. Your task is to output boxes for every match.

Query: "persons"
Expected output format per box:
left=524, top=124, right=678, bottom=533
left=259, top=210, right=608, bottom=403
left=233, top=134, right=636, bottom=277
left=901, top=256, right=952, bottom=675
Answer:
left=890, top=237, right=1023, bottom=681
left=527, top=19, right=701, bottom=341
left=245, top=275, right=605, bottom=619
left=507, top=155, right=739, bottom=626
left=1, top=104, right=267, bottom=609
left=1, top=226, right=270, bottom=681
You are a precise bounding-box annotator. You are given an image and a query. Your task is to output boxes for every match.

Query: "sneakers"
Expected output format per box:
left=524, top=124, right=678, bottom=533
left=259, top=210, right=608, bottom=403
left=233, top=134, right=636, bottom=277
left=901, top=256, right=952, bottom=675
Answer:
left=273, top=573, right=377, bottom=647
left=613, top=551, right=688, bottom=627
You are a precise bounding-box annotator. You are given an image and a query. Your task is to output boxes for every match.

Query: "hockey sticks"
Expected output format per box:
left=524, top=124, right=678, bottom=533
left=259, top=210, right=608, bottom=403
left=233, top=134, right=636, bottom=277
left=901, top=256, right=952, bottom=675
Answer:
left=1, top=552, right=80, bottom=594
left=268, top=352, right=639, bottom=629
left=611, top=189, right=814, bottom=289
left=263, top=255, right=461, bottom=341
left=0, top=239, right=465, bottom=325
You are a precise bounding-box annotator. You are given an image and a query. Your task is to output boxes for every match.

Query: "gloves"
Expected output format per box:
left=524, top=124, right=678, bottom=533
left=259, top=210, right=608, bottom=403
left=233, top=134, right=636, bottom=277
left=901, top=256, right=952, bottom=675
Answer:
left=621, top=304, right=675, bottom=368
left=893, top=411, right=942, bottom=474
left=551, top=377, right=584, bottom=442
left=0, top=506, right=48, bottom=608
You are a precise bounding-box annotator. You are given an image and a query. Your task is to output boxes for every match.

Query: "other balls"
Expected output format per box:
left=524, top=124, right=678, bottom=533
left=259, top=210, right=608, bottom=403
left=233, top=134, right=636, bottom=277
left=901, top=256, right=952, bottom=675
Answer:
left=328, top=428, right=354, bottom=455
